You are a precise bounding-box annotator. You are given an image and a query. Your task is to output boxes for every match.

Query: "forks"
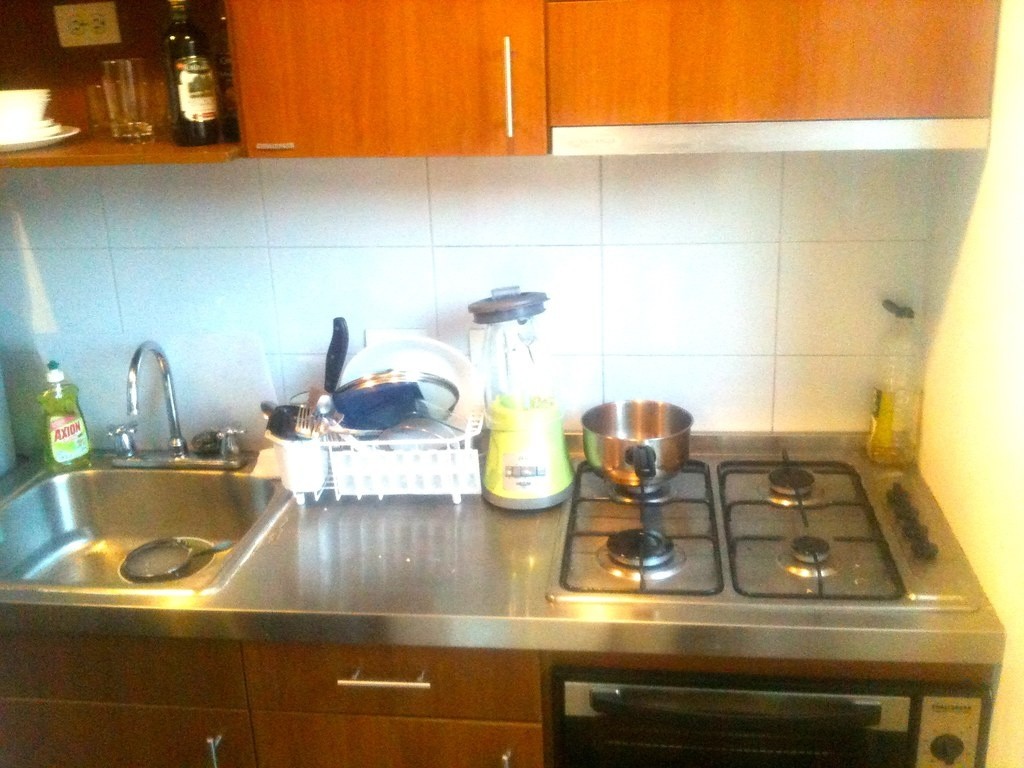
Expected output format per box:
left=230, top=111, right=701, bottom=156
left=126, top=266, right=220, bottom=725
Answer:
left=294, top=405, right=313, bottom=440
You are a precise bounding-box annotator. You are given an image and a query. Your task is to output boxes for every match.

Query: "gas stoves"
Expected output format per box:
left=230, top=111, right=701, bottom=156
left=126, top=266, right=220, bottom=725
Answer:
left=543, top=456, right=984, bottom=619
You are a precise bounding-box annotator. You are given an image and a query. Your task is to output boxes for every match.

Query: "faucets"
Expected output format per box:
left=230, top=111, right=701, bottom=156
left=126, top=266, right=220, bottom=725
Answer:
left=106, top=339, right=249, bottom=469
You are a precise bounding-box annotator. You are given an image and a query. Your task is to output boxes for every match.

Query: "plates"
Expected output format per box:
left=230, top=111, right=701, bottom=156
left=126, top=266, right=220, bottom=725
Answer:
left=0, top=89, right=80, bottom=151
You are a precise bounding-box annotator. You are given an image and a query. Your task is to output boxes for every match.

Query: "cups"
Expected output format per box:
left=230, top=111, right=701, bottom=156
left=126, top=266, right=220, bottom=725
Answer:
left=83, top=54, right=166, bottom=145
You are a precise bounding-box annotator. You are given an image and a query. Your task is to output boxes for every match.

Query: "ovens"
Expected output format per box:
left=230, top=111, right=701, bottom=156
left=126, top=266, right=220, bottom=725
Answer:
left=550, top=667, right=994, bottom=768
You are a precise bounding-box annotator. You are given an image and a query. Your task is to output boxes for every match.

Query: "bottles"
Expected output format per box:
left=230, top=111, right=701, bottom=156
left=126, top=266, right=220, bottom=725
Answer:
left=168, top=0, right=220, bottom=147
left=866, top=300, right=925, bottom=466
left=37, top=360, right=92, bottom=473
left=217, top=14, right=240, bottom=143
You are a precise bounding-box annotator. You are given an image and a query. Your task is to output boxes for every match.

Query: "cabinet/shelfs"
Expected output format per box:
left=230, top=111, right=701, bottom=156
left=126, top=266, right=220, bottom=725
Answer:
left=0, top=630, right=556, bottom=768
left=0, top=0, right=1002, bottom=167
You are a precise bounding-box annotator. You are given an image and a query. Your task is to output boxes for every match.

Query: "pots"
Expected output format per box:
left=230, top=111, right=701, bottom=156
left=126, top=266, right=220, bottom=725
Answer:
left=581, top=400, right=695, bottom=486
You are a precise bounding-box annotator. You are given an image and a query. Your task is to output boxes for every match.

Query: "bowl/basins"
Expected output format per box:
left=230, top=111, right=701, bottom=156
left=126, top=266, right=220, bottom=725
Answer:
left=117, top=534, right=217, bottom=583
left=123, top=538, right=193, bottom=581
left=335, top=369, right=461, bottom=422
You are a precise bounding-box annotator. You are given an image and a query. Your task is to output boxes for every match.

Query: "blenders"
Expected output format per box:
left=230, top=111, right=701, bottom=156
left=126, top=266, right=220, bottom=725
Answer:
left=468, top=284, right=575, bottom=510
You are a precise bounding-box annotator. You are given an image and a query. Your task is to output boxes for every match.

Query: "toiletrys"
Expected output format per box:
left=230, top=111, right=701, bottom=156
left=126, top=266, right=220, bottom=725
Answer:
left=35, top=360, right=96, bottom=475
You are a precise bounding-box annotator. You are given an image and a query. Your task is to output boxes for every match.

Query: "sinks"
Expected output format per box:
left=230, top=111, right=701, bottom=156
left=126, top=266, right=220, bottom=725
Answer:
left=0, top=449, right=293, bottom=596
